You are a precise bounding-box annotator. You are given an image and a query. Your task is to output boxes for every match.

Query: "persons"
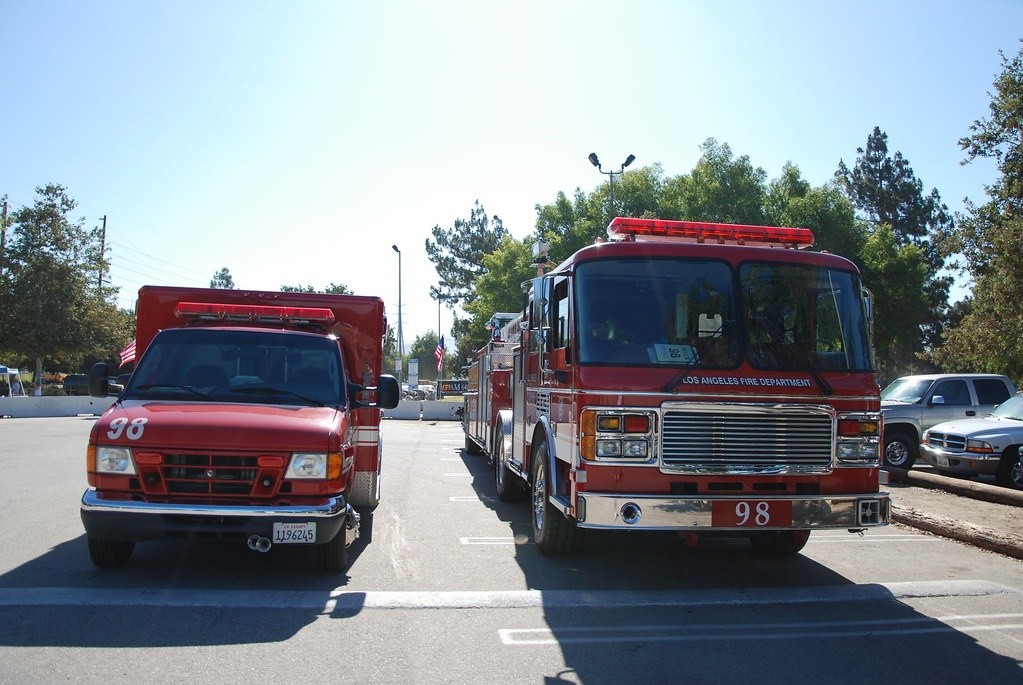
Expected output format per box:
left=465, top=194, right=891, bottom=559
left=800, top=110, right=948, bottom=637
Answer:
left=12, top=378, right=20, bottom=395
left=4, top=383, right=9, bottom=397
left=30, top=382, right=41, bottom=396
left=588, top=313, right=644, bottom=352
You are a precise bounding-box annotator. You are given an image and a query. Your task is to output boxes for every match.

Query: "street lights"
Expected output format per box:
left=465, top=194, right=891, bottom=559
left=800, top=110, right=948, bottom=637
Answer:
left=392, top=243, right=402, bottom=402
left=588, top=152, right=637, bottom=242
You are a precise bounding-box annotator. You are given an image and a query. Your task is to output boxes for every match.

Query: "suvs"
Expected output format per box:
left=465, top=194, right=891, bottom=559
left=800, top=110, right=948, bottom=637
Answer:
left=63, top=373, right=125, bottom=396
left=881, top=372, right=1020, bottom=480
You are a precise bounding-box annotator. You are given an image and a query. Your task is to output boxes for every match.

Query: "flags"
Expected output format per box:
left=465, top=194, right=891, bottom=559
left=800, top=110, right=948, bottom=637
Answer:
left=435, top=336, right=444, bottom=372
left=119, top=340, right=135, bottom=368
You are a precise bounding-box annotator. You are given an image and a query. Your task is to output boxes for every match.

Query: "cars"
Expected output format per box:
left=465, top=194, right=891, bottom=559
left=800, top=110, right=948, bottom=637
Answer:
left=398, top=380, right=439, bottom=401
left=918, top=392, right=1023, bottom=492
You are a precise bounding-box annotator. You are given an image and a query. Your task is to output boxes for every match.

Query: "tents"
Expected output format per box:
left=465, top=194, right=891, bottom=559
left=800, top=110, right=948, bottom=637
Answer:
left=0, top=365, right=26, bottom=397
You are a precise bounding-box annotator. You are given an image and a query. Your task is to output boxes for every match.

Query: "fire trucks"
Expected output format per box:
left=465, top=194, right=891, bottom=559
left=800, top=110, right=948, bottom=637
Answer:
left=78, top=285, right=402, bottom=577
left=459, top=216, right=895, bottom=560
